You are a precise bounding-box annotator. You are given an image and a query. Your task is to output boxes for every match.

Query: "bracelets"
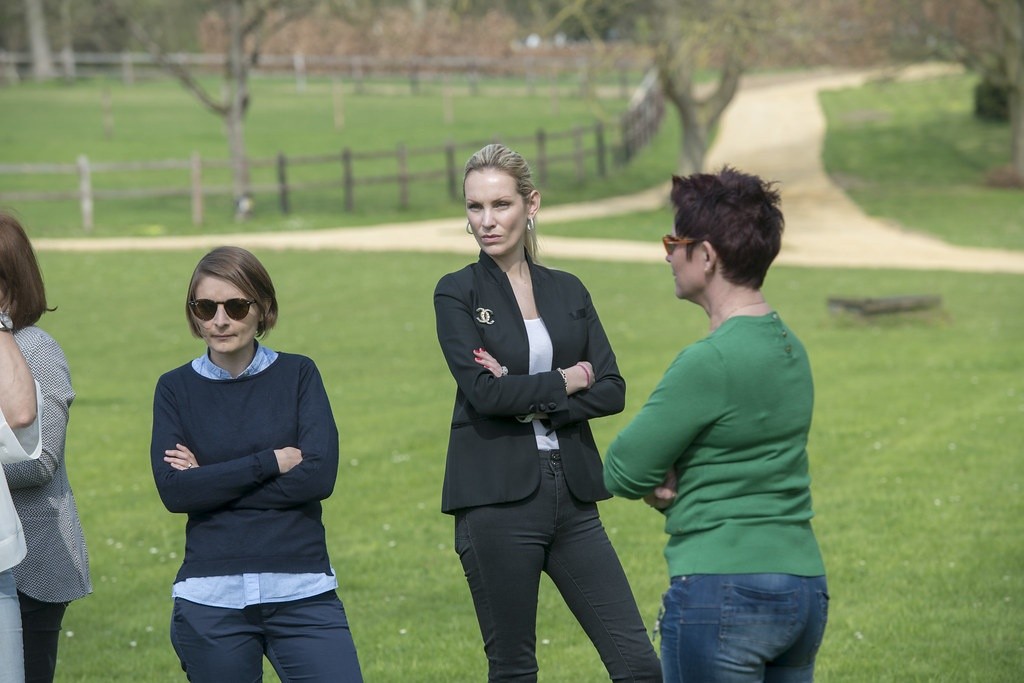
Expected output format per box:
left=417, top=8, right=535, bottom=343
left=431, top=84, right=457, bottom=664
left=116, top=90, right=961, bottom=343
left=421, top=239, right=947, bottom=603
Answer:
left=556, top=368, right=568, bottom=391
left=577, top=363, right=590, bottom=388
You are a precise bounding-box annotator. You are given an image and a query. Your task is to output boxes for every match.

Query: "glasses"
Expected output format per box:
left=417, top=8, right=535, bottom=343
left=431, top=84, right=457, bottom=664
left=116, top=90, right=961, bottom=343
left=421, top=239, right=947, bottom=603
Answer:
left=663, top=234, right=701, bottom=254
left=184, top=296, right=258, bottom=320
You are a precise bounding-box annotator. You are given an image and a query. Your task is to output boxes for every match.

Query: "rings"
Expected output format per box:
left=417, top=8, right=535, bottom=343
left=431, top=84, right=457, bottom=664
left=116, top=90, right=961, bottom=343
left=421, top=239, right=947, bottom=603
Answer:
left=501, top=366, right=508, bottom=374
left=188, top=463, right=192, bottom=468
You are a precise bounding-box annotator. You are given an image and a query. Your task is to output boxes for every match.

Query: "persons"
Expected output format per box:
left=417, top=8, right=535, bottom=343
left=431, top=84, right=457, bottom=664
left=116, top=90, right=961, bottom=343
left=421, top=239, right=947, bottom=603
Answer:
left=0, top=213, right=92, bottom=683
left=150, top=246, right=364, bottom=683
left=433, top=142, right=663, bottom=683
left=603, top=167, right=830, bottom=683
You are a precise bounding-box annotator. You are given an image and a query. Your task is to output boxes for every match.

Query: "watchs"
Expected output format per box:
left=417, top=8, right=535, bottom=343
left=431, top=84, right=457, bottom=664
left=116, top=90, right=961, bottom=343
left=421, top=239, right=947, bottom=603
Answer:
left=0, top=311, right=14, bottom=331
